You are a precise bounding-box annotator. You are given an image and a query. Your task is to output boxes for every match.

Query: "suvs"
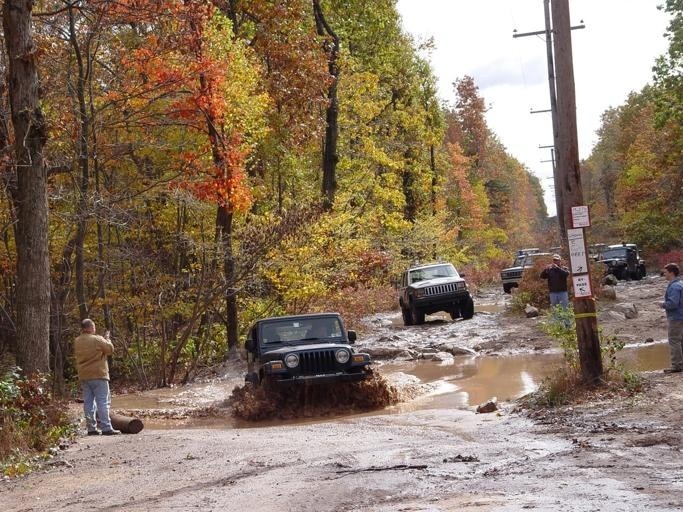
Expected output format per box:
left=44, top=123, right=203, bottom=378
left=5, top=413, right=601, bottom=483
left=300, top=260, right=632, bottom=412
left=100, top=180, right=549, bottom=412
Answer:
left=501, top=253, right=557, bottom=296
left=399, top=262, right=474, bottom=325
left=242, top=309, right=373, bottom=408
left=586, top=242, right=647, bottom=281
left=516, top=246, right=539, bottom=254
left=548, top=246, right=564, bottom=254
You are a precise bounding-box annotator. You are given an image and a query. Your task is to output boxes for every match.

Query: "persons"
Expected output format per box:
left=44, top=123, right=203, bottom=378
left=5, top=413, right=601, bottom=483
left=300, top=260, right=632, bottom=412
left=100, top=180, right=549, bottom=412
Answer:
left=73, top=318, right=121, bottom=435
left=653, top=263, right=683, bottom=373
left=538, top=254, right=572, bottom=326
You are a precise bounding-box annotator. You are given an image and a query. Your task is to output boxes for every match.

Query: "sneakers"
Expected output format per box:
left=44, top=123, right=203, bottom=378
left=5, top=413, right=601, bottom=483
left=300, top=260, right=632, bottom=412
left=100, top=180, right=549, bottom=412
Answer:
left=88, top=430, right=102, bottom=435
left=102, top=429, right=121, bottom=434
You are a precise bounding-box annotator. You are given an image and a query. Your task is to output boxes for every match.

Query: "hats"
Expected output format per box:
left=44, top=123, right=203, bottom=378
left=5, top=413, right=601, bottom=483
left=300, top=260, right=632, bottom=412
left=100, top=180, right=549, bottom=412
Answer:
left=553, top=255, right=561, bottom=260
